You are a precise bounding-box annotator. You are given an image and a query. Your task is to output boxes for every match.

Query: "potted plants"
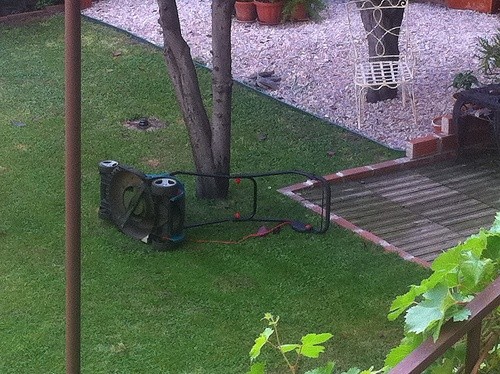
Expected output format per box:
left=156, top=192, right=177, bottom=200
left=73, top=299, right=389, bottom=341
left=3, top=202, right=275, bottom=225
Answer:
left=235, top=0, right=326, bottom=23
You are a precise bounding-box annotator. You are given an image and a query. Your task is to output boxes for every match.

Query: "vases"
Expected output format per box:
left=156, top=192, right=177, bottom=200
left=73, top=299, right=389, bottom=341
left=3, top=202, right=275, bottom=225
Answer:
left=432, top=116, right=442, bottom=134
